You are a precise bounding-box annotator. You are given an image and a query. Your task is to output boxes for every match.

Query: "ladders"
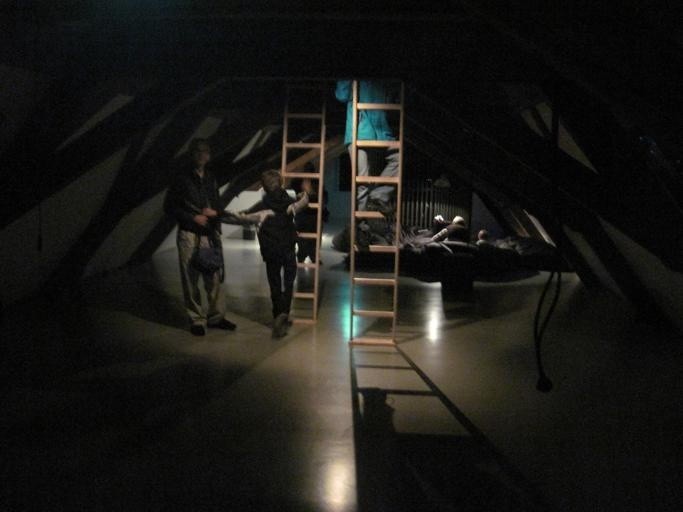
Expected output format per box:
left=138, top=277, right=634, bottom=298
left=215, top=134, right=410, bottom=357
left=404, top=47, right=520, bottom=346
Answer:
left=348, top=80, right=403, bottom=347
left=279, top=95, right=324, bottom=322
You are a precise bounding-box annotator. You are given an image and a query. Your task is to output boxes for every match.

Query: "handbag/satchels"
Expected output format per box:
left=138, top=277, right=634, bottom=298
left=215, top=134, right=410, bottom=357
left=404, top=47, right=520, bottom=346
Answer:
left=192, top=248, right=220, bottom=273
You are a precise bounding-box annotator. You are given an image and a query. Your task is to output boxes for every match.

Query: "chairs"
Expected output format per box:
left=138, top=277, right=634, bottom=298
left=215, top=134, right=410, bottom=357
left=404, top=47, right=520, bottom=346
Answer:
left=419, top=241, right=479, bottom=283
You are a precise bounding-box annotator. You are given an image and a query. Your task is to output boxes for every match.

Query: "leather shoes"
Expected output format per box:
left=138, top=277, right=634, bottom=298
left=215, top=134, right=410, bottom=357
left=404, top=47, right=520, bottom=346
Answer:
left=207, top=317, right=237, bottom=331
left=191, top=324, right=205, bottom=336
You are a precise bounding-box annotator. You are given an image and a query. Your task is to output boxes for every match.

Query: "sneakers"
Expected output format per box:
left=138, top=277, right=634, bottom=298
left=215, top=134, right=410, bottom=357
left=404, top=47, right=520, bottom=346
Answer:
left=357, top=223, right=369, bottom=247
left=272, top=312, right=290, bottom=339
left=366, top=196, right=394, bottom=214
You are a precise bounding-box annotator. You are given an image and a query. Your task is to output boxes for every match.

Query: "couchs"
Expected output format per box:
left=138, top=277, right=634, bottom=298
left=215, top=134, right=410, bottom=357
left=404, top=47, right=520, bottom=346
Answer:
left=333, top=228, right=547, bottom=281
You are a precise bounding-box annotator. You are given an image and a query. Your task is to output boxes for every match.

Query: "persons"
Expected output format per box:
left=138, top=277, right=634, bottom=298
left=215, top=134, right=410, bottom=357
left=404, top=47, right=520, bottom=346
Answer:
left=240, top=172, right=309, bottom=336
left=334, top=81, right=409, bottom=245
left=290, top=161, right=319, bottom=263
left=413, top=215, right=490, bottom=249
left=162, top=137, right=237, bottom=334
